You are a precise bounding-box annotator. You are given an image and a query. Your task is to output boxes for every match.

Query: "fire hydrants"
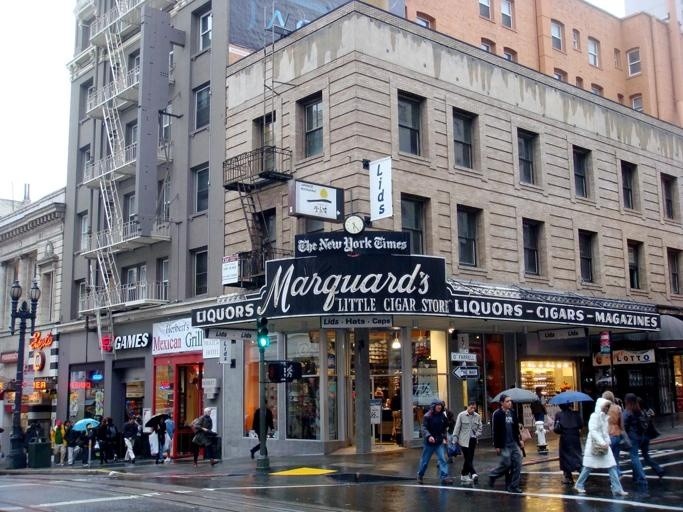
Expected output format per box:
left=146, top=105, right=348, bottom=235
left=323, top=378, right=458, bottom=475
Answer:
left=533, top=421, right=550, bottom=455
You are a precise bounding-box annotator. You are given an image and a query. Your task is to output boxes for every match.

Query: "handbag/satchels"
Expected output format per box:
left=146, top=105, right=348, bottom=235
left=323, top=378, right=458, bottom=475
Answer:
left=591, top=442, right=608, bottom=458
left=645, top=411, right=660, bottom=439
left=618, top=430, right=632, bottom=451
left=553, top=413, right=562, bottom=434
left=518, top=423, right=534, bottom=441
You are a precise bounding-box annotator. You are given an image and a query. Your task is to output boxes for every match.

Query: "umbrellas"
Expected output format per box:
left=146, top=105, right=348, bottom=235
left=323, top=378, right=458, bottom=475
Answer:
left=489, top=388, right=540, bottom=416
left=549, top=391, right=594, bottom=410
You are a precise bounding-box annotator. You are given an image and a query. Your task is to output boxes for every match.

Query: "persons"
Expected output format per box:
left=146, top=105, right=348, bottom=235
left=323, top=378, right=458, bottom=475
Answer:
left=554, top=401, right=589, bottom=484
left=250, top=398, right=273, bottom=458
left=602, top=390, right=663, bottom=498
left=417, top=397, right=456, bottom=486
left=572, top=397, right=629, bottom=495
left=531, top=386, right=548, bottom=419
left=192, top=408, right=218, bottom=465
left=488, top=394, right=524, bottom=493
left=390, top=387, right=401, bottom=438
left=505, top=400, right=527, bottom=485
left=24, top=409, right=175, bottom=467
left=452, top=401, right=482, bottom=482
left=374, top=385, right=388, bottom=404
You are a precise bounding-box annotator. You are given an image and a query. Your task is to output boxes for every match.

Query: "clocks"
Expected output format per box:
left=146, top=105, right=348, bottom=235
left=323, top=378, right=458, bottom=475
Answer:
left=344, top=215, right=365, bottom=235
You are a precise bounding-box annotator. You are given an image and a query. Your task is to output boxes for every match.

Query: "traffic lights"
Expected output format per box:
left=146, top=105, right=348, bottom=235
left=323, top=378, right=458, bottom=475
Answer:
left=257, top=315, right=269, bottom=350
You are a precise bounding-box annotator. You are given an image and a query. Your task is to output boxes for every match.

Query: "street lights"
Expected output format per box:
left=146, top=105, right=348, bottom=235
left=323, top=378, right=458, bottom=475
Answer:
left=8, top=277, right=41, bottom=471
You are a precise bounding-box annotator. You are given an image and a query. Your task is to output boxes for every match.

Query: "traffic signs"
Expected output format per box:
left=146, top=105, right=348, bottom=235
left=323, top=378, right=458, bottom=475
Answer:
left=453, top=366, right=481, bottom=380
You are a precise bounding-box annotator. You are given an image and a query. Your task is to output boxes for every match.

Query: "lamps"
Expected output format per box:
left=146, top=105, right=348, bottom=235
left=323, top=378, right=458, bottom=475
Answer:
left=413, top=320, right=419, bottom=329
left=389, top=327, right=402, bottom=349
left=450, top=321, right=456, bottom=331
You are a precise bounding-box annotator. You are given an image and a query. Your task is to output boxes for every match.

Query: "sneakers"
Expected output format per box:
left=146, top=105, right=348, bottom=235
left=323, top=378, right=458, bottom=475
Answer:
left=414, top=469, right=667, bottom=497
left=53, top=449, right=266, bottom=468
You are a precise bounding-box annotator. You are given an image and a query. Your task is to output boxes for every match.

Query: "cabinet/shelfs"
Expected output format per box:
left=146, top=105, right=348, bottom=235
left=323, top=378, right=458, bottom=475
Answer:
left=520, top=370, right=556, bottom=397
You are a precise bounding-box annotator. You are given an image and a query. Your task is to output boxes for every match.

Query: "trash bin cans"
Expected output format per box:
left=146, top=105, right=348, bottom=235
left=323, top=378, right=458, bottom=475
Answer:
left=27, top=437, right=51, bottom=468
